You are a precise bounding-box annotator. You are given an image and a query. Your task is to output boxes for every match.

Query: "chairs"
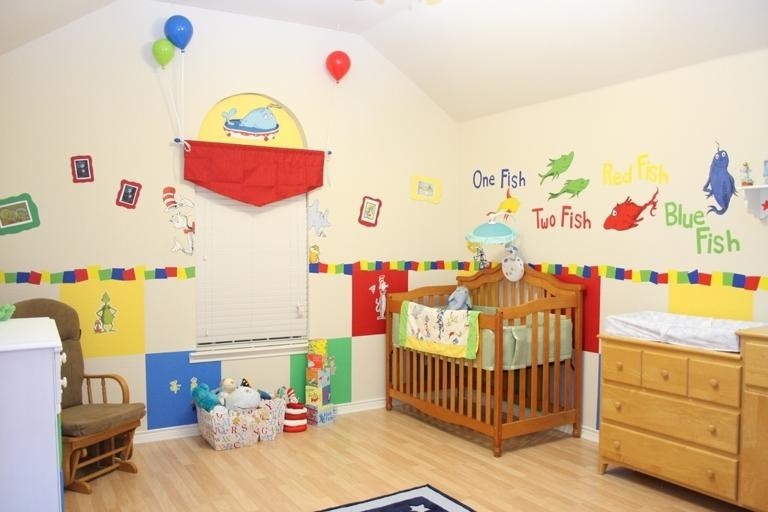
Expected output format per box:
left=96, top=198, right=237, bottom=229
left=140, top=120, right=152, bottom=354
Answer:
left=9, top=298, right=149, bottom=494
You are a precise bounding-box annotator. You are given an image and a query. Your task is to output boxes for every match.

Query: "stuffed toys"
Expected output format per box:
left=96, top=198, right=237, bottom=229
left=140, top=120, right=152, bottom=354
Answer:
left=447, top=286, right=473, bottom=310
left=191, top=377, right=286, bottom=414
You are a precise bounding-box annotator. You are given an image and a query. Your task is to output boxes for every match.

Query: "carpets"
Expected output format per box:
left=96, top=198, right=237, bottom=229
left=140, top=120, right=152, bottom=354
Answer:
left=313, top=482, right=477, bottom=512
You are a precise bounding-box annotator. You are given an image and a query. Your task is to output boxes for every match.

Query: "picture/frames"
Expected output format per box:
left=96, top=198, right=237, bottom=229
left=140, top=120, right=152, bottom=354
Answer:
left=0, top=193, right=41, bottom=236
left=70, top=155, right=95, bottom=184
left=409, top=174, right=442, bottom=205
left=115, top=179, right=142, bottom=209
left=357, top=196, right=382, bottom=227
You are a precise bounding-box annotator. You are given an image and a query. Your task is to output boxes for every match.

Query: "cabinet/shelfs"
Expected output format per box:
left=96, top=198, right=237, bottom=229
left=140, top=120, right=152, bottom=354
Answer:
left=0, top=316, right=69, bottom=512
left=595, top=324, right=767, bottom=511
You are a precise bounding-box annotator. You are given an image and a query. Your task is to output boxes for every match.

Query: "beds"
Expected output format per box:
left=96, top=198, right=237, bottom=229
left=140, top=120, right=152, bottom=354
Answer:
left=384, top=261, right=585, bottom=457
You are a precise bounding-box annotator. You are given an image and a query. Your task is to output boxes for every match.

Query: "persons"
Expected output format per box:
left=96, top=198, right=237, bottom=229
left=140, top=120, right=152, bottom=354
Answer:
left=283, top=387, right=307, bottom=433
left=125, top=189, right=134, bottom=204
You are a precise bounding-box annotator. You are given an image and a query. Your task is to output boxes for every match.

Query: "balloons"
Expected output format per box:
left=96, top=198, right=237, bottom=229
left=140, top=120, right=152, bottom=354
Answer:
left=325, top=50, right=352, bottom=84
left=163, top=15, right=194, bottom=54
left=151, top=39, right=175, bottom=70
